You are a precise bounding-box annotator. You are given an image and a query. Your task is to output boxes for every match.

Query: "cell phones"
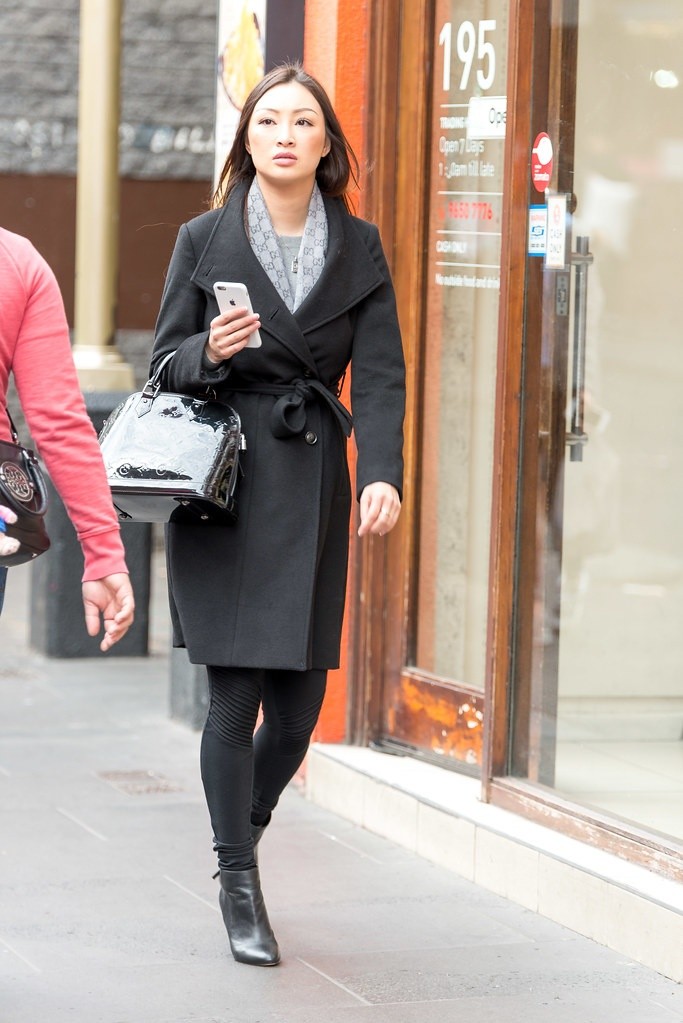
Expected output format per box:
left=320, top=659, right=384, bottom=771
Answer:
left=213, top=281, right=262, bottom=348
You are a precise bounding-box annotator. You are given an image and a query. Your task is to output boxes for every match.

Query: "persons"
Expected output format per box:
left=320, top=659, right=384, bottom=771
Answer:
left=150, top=65, right=404, bottom=967
left=0, top=227, right=135, bottom=652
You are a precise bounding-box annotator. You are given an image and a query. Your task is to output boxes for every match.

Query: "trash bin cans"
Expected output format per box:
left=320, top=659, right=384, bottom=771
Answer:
left=29, top=394, right=151, bottom=657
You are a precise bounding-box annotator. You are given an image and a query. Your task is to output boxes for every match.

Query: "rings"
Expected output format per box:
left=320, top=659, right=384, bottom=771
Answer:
left=381, top=509, right=391, bottom=518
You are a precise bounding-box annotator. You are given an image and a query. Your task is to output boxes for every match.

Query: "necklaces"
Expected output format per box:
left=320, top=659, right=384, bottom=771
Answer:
left=278, top=231, right=299, bottom=273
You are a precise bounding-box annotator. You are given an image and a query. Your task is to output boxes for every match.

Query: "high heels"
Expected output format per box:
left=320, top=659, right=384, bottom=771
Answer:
left=212, top=865, right=281, bottom=966
left=250, top=810, right=272, bottom=866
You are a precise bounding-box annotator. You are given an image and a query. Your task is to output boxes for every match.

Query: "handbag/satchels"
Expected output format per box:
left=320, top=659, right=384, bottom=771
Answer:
left=97, top=349, right=247, bottom=528
left=0, top=439, right=51, bottom=569
